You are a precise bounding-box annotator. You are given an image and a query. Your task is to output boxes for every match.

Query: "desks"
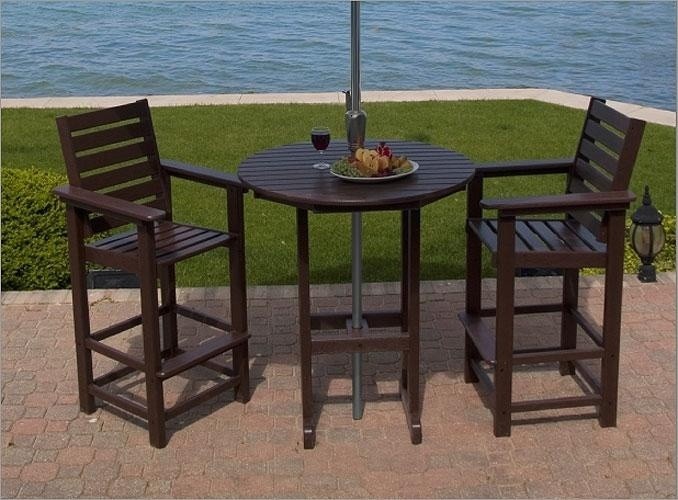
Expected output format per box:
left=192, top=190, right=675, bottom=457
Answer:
left=237, top=139, right=476, bottom=450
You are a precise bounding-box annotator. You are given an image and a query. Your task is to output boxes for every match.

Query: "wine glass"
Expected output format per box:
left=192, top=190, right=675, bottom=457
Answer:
left=311, top=126, right=332, bottom=170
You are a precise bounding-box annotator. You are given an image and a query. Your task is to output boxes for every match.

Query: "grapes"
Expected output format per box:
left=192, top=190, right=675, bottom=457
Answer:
left=332, top=159, right=361, bottom=177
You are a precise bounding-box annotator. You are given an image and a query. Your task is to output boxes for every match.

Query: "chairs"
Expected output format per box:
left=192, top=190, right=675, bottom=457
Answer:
left=457, top=94, right=646, bottom=441
left=49, top=99, right=251, bottom=447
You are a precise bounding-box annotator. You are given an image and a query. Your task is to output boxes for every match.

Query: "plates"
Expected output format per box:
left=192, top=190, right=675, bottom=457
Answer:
left=330, top=160, right=419, bottom=183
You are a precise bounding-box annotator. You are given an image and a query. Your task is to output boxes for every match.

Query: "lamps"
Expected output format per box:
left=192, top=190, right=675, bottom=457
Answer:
left=630, top=185, right=664, bottom=282
